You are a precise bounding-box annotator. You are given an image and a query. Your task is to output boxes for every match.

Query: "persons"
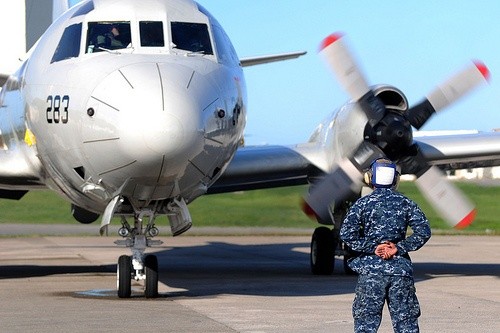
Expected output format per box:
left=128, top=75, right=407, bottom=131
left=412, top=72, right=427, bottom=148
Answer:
left=341, top=160, right=431, bottom=333
left=91, top=24, right=127, bottom=51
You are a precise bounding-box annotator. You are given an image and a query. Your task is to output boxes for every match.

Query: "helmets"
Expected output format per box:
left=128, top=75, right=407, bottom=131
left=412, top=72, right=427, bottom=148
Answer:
left=370, top=158, right=397, bottom=187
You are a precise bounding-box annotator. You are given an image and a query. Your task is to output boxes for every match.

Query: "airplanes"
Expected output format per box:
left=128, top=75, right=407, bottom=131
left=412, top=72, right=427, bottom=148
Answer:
left=0, top=0, right=500, bottom=300
